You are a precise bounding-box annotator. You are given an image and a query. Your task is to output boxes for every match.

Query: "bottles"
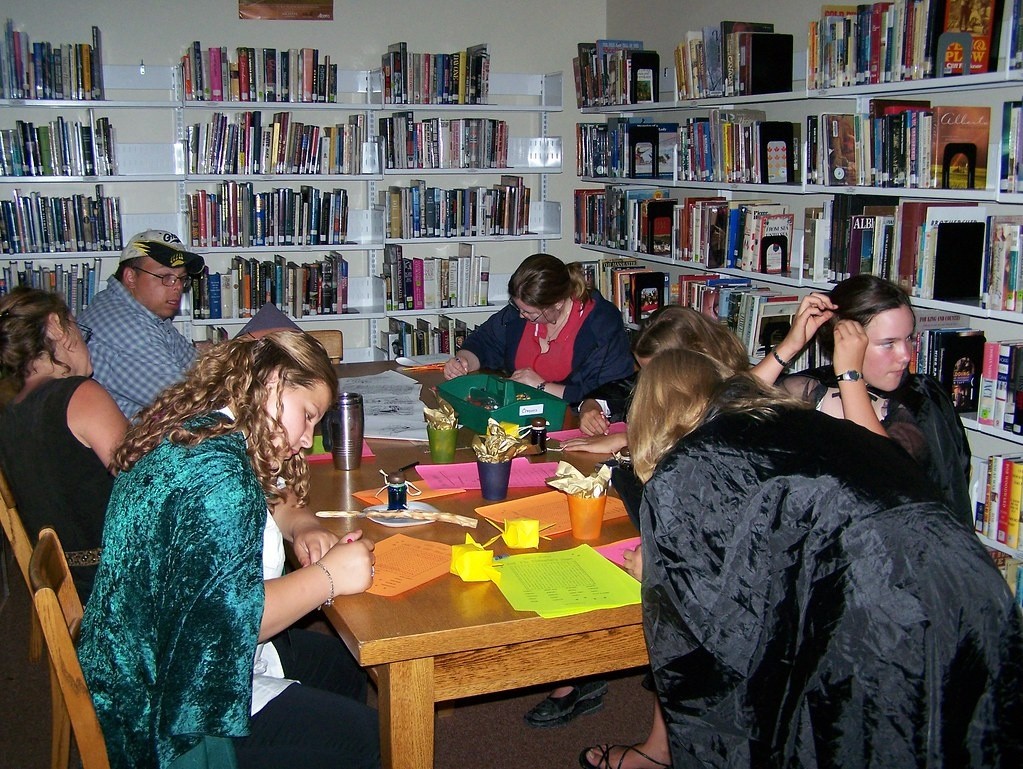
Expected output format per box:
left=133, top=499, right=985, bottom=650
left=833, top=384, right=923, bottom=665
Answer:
left=620, top=446, right=634, bottom=472
left=531, top=417, right=547, bottom=456
left=386, top=472, right=407, bottom=510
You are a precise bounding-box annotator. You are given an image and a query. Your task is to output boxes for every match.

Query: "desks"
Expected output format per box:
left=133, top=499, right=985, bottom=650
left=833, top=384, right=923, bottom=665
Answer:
left=234, top=357, right=649, bottom=769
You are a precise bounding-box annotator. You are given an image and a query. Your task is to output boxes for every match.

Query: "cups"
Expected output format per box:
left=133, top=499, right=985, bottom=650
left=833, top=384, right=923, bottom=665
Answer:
left=568, top=494, right=607, bottom=540
left=427, top=425, right=458, bottom=464
left=477, top=461, right=511, bottom=501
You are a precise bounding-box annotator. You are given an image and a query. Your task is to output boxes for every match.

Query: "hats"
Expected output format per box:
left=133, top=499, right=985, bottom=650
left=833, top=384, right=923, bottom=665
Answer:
left=119, top=228, right=205, bottom=275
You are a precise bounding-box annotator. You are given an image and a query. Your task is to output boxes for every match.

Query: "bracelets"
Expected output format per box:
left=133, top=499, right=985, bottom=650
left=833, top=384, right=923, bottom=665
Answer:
left=315, top=562, right=335, bottom=611
left=771, top=344, right=792, bottom=366
left=537, top=381, right=549, bottom=392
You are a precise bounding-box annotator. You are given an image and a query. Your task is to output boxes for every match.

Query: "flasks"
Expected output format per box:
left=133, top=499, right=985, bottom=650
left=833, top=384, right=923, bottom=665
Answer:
left=322, top=393, right=364, bottom=470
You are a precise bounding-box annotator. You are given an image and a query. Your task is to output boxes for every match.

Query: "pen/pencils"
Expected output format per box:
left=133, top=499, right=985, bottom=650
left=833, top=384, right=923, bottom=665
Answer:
left=399, top=461, right=420, bottom=471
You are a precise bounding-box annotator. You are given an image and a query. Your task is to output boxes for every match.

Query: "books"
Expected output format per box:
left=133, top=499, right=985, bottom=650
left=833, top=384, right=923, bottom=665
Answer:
left=0, top=0, right=1022, bottom=613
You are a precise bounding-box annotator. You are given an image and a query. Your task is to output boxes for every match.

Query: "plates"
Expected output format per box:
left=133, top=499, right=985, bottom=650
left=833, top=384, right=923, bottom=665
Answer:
left=365, top=501, right=440, bottom=527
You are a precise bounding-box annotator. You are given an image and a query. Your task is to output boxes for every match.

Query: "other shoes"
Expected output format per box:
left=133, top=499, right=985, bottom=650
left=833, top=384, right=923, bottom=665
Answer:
left=523, top=695, right=604, bottom=729
left=532, top=674, right=608, bottom=721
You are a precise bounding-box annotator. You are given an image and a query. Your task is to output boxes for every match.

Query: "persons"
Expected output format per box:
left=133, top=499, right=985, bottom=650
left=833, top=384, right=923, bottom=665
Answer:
left=443, top=253, right=634, bottom=405
left=523, top=306, right=750, bottom=729
left=625, top=347, right=1023, bottom=769
left=579, top=274, right=973, bottom=769
left=0, top=286, right=132, bottom=607
left=75, top=230, right=209, bottom=427
left=77, top=301, right=381, bottom=769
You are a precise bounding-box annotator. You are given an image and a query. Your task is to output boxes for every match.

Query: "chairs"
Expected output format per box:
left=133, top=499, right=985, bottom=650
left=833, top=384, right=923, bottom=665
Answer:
left=1, top=458, right=112, bottom=769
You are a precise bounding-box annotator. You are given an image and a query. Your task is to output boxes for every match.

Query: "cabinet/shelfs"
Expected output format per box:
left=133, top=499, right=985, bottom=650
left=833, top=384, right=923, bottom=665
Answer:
left=0, top=66, right=567, bottom=374
left=576, top=32, right=1022, bottom=604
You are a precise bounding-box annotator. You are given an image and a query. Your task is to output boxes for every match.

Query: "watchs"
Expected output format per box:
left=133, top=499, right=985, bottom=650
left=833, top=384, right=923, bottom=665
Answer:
left=836, top=370, right=864, bottom=382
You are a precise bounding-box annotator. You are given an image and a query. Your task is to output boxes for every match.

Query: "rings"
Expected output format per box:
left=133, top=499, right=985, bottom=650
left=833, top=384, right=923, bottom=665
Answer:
left=370, top=566, right=374, bottom=577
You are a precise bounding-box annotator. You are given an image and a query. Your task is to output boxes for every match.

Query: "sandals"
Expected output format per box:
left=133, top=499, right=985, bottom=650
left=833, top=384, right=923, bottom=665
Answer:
left=579, top=743, right=673, bottom=769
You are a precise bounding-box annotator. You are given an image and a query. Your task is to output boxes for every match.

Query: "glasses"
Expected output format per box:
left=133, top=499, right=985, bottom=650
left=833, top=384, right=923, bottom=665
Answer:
left=63, top=319, right=92, bottom=344
left=508, top=296, right=550, bottom=322
left=133, top=266, right=192, bottom=288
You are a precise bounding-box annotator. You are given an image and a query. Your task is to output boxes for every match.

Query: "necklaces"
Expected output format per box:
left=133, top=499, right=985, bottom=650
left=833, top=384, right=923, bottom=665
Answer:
left=547, top=300, right=571, bottom=345
